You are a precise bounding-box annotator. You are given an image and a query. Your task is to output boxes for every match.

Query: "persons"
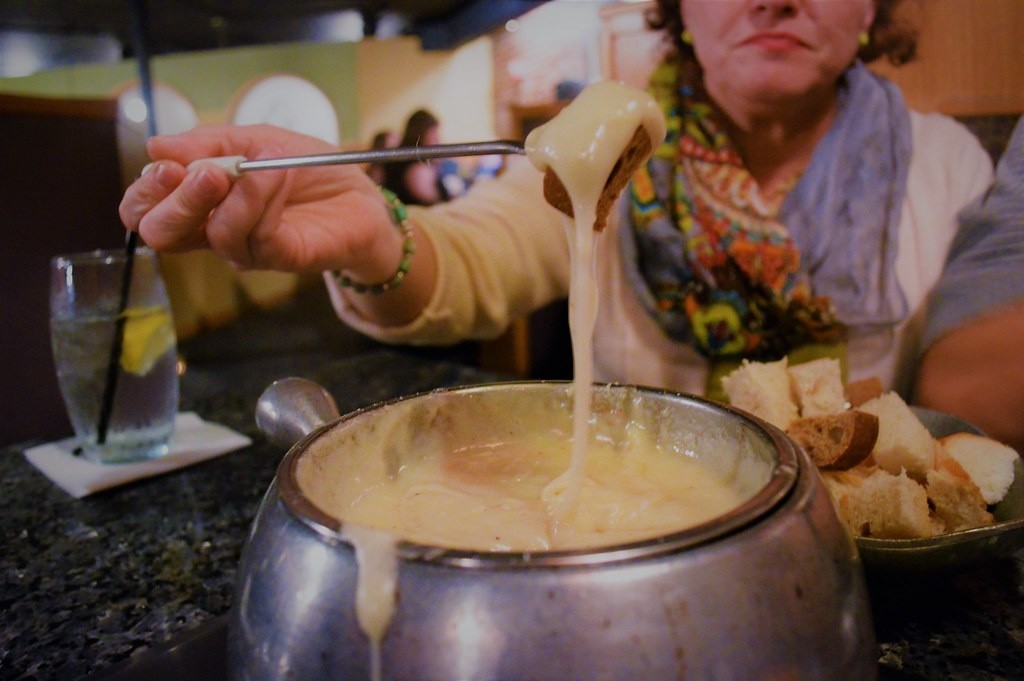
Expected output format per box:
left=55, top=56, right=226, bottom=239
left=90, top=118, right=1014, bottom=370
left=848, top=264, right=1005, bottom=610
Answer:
left=364, top=128, right=398, bottom=188
left=911, top=97, right=1019, bottom=454
left=385, top=105, right=444, bottom=205
left=115, top=0, right=1001, bottom=426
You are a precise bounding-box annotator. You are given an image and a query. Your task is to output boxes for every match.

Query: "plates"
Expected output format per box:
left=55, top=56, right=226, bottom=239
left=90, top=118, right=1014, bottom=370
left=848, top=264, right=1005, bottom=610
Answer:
left=855, top=404, right=1023, bottom=572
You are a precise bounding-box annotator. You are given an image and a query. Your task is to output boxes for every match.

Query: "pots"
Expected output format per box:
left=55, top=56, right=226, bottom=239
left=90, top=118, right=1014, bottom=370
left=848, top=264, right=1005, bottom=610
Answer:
left=232, top=374, right=867, bottom=679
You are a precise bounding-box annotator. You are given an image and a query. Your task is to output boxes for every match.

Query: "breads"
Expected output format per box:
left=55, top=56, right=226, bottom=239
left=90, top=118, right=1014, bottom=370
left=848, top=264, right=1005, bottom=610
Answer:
left=721, top=356, right=1022, bottom=540
left=544, top=79, right=666, bottom=232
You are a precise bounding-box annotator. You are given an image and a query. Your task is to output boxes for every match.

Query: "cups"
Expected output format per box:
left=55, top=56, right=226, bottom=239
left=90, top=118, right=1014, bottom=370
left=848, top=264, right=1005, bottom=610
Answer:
left=49, top=246, right=181, bottom=468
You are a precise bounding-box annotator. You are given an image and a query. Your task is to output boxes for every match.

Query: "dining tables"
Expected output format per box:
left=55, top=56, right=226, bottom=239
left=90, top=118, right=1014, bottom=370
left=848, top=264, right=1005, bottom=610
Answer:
left=0, top=350, right=1024, bottom=680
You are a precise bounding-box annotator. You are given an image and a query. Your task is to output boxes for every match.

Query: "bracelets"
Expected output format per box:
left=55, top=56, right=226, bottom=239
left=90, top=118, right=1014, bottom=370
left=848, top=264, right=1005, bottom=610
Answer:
left=333, top=176, right=418, bottom=297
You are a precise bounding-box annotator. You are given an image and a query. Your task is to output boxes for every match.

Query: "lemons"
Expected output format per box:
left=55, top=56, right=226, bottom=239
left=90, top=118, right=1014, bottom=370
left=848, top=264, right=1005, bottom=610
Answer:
left=120, top=307, right=176, bottom=378
